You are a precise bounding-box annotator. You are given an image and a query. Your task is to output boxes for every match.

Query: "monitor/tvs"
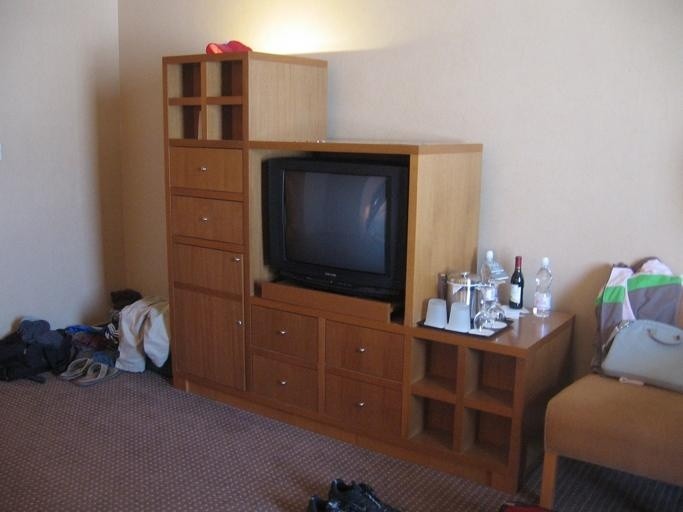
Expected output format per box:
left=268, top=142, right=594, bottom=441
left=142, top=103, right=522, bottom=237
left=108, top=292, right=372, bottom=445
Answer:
left=265, top=156, right=409, bottom=302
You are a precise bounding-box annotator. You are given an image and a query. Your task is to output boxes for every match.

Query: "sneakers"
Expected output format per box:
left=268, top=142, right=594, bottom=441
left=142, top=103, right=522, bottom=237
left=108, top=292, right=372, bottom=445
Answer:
left=307, top=495, right=351, bottom=511
left=328, top=479, right=400, bottom=511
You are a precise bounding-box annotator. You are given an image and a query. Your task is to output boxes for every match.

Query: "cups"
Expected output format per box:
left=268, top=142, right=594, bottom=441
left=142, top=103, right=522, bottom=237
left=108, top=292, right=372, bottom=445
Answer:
left=425, top=299, right=447, bottom=328
left=447, top=303, right=471, bottom=332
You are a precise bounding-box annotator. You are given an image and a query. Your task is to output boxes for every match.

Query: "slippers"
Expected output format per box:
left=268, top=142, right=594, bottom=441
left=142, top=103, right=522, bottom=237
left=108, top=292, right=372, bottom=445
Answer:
left=74, top=363, right=120, bottom=386
left=59, top=358, right=96, bottom=380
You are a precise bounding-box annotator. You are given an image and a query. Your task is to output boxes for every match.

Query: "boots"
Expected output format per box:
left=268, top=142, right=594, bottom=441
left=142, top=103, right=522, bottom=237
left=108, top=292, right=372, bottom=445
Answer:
left=97, top=289, right=142, bottom=351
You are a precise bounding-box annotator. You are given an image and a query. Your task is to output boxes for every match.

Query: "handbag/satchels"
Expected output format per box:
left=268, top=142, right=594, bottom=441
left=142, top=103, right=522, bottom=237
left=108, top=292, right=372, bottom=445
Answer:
left=600, top=320, right=683, bottom=393
left=0, top=328, right=71, bottom=383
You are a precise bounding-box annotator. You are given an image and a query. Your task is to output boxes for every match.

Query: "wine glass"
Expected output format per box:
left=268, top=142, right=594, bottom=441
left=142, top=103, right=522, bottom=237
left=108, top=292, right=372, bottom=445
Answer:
left=474, top=279, right=506, bottom=334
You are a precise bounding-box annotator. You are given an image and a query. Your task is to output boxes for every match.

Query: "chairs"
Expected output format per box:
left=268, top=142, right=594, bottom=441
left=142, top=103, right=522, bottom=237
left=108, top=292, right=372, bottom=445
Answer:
left=538, top=270, right=682, bottom=512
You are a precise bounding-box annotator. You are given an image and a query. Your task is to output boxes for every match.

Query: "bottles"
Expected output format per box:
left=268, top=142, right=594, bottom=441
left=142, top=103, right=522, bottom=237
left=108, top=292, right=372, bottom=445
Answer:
left=509, top=256, right=524, bottom=309
left=533, top=257, right=553, bottom=318
left=481, top=251, right=498, bottom=304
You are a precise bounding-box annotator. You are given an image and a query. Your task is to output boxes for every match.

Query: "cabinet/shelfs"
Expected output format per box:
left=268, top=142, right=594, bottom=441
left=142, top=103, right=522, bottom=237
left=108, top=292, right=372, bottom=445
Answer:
left=162, top=52, right=575, bottom=494
